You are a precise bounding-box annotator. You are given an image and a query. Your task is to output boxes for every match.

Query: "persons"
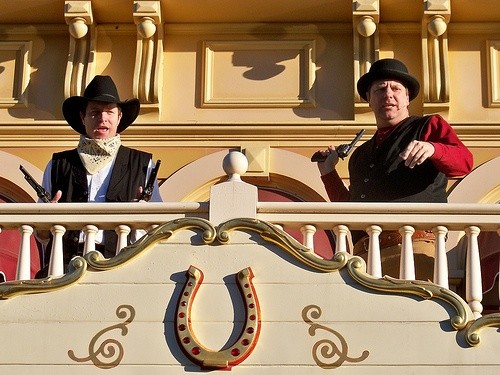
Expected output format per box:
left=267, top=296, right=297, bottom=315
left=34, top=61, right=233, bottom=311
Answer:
left=317, top=58, right=474, bottom=283
left=32, top=75, right=163, bottom=279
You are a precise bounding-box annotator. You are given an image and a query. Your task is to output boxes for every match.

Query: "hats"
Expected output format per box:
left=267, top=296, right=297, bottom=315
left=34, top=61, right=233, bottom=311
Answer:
left=62, top=75, right=140, bottom=138
left=357, top=59, right=421, bottom=103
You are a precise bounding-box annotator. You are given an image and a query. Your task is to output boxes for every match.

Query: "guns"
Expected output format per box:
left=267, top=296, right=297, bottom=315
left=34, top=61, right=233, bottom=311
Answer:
left=138, top=159, right=161, bottom=201
left=311, top=129, right=365, bottom=162
left=19, top=165, right=52, bottom=203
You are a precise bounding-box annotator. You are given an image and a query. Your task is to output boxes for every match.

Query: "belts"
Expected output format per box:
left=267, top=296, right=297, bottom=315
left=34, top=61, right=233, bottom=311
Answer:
left=45, top=252, right=83, bottom=266
left=353, top=228, right=448, bottom=257
